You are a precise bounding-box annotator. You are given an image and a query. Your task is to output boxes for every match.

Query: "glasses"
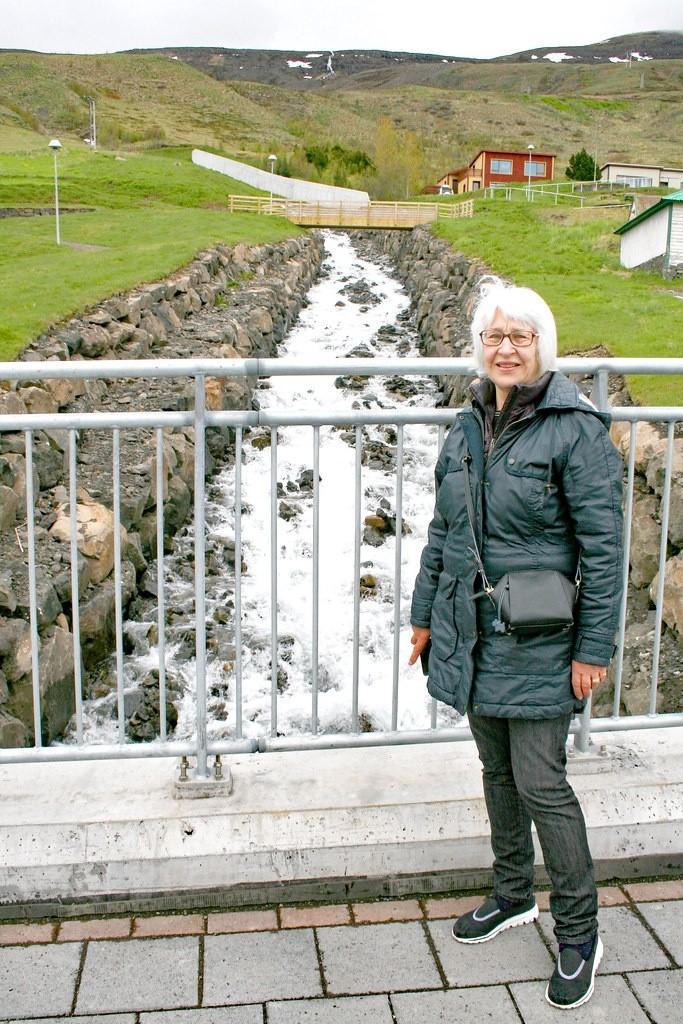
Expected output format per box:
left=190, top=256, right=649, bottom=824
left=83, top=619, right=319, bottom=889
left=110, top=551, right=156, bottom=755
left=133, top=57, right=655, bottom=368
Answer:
left=478, top=329, right=540, bottom=347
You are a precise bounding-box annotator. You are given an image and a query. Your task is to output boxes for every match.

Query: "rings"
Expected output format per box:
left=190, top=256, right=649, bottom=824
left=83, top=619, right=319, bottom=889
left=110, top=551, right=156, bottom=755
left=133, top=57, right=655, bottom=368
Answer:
left=592, top=677, right=600, bottom=682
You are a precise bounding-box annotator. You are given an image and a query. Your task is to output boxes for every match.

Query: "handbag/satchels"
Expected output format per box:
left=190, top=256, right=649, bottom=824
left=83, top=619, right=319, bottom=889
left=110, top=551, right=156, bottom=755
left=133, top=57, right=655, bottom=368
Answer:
left=487, top=569, right=576, bottom=629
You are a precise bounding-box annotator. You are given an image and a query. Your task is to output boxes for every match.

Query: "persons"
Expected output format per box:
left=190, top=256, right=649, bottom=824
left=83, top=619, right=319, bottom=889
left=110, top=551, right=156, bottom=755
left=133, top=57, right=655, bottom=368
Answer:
left=409, top=274, right=625, bottom=1010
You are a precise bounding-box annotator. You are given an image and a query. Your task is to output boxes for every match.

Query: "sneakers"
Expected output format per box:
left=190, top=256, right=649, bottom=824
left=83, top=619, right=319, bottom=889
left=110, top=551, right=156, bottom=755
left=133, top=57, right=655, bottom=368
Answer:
left=544, top=934, right=604, bottom=1010
left=451, top=890, right=539, bottom=944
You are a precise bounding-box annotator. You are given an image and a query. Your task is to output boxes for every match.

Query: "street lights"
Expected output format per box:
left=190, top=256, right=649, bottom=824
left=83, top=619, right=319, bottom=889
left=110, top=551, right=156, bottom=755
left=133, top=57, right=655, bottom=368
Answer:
left=527, top=144, right=535, bottom=202
left=47, top=140, right=62, bottom=245
left=268, top=154, right=278, bottom=216
